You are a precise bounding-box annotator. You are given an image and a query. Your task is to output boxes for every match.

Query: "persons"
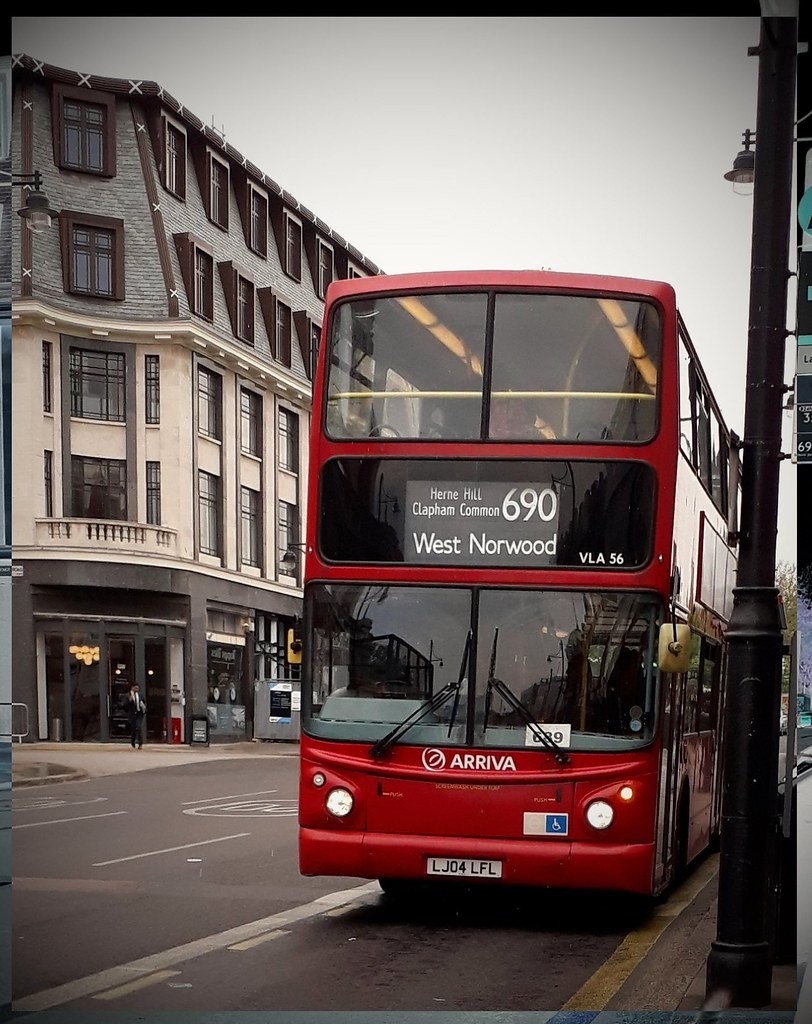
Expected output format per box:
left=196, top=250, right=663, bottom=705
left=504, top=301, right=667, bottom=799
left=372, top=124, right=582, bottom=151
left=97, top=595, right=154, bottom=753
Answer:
left=329, top=665, right=390, bottom=700
left=122, top=684, right=147, bottom=749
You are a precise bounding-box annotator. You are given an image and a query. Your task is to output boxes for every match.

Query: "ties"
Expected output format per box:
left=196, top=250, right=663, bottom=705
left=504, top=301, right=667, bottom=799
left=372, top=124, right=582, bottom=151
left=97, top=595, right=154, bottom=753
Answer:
left=134, top=692, right=137, bottom=706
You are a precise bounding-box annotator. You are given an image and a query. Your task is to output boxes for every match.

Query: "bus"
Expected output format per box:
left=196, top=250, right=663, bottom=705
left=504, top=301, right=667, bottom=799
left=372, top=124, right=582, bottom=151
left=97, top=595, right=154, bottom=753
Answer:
left=286, top=268, right=735, bottom=900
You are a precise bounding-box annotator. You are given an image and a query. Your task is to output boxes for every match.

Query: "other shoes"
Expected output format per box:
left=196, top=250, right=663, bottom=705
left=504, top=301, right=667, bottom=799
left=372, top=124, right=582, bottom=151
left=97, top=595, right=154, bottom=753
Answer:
left=138, top=744, right=142, bottom=749
left=131, top=741, right=135, bottom=747
